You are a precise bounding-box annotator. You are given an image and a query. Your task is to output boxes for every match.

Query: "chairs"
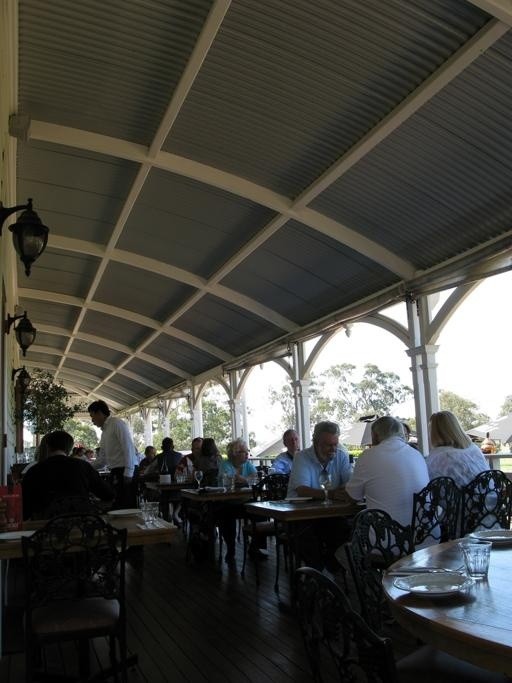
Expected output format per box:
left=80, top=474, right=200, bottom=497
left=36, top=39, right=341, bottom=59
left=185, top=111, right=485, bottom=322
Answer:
left=291, top=567, right=483, bottom=682
left=462, top=469, right=512, bottom=535
left=22, top=512, right=128, bottom=680
left=412, top=476, right=460, bottom=547
left=344, top=508, right=415, bottom=630
left=242, top=473, right=289, bottom=584
left=1, top=449, right=271, bottom=514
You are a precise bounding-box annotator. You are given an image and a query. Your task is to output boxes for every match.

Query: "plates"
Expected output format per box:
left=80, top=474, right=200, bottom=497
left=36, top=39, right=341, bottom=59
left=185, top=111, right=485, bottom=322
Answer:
left=285, top=496, right=313, bottom=504
left=106, top=508, right=142, bottom=517
left=467, top=530, right=511, bottom=544
left=0, top=530, right=36, bottom=542
left=393, top=572, right=475, bottom=597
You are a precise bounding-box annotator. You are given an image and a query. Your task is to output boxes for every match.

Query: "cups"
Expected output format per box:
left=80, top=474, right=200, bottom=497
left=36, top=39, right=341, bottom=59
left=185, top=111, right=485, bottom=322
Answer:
left=140, top=500, right=160, bottom=524
left=222, top=474, right=234, bottom=488
left=463, top=582, right=499, bottom=619
left=17, top=447, right=36, bottom=464
left=458, top=540, right=489, bottom=582
left=268, top=468, right=275, bottom=476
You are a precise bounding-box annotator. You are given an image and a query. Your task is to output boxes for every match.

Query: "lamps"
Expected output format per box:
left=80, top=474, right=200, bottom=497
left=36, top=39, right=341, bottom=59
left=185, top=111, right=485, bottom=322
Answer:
left=2, top=308, right=39, bottom=359
left=0, top=194, right=52, bottom=279
left=10, top=362, right=32, bottom=392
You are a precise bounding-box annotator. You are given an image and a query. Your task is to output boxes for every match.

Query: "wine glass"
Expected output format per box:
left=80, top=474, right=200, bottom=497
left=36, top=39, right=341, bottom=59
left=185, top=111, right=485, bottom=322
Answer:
left=318, top=472, right=333, bottom=505
left=195, top=471, right=204, bottom=489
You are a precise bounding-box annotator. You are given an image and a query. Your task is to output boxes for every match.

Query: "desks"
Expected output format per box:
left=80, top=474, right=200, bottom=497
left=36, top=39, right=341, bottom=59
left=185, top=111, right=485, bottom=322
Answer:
left=246, top=497, right=367, bottom=521
left=2, top=512, right=177, bottom=558
left=383, top=531, right=512, bottom=679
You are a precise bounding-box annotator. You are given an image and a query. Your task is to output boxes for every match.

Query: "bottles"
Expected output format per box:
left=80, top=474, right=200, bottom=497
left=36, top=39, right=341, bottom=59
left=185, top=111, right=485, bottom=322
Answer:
left=256, top=471, right=266, bottom=491
left=160, top=454, right=171, bottom=485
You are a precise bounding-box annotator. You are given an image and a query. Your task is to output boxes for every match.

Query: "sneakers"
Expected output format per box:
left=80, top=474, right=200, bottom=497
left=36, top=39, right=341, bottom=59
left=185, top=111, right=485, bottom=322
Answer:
left=187, top=545, right=268, bottom=568
left=155, top=510, right=184, bottom=526
left=356, top=593, right=399, bottom=629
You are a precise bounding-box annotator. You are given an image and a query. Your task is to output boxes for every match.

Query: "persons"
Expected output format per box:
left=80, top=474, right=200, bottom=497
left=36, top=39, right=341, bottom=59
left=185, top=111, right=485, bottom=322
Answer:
left=423, top=409, right=503, bottom=538
left=80, top=398, right=141, bottom=559
left=286, top=420, right=354, bottom=574
left=401, top=421, right=421, bottom=452
left=18, top=427, right=303, bottom=561
left=343, top=415, right=442, bottom=558
